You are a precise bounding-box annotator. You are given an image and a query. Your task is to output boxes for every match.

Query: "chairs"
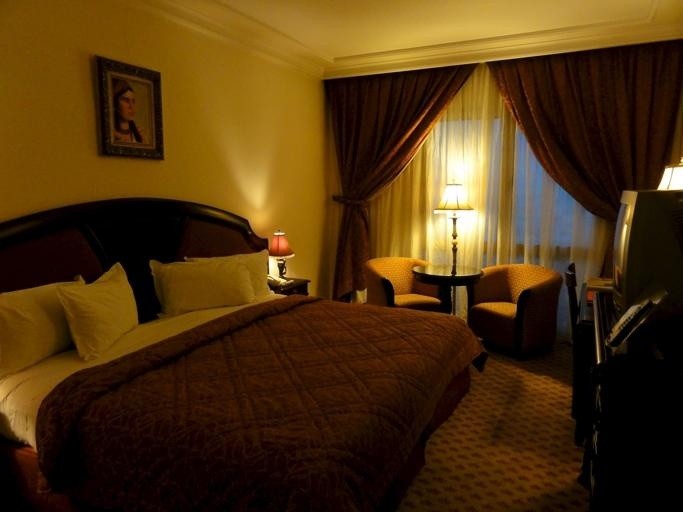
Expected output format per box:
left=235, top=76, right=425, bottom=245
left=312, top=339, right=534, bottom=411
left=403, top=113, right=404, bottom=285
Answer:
left=364, top=256, right=451, bottom=313
left=565, top=262, right=595, bottom=446
left=467, top=264, right=563, bottom=352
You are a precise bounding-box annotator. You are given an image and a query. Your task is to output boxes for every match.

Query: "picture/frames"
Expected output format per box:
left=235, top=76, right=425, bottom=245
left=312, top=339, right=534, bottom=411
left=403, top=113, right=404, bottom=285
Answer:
left=93, top=54, right=165, bottom=161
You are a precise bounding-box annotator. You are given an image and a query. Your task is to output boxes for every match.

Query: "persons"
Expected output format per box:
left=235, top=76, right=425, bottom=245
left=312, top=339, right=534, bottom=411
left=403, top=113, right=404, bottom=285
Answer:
left=111, top=77, right=144, bottom=143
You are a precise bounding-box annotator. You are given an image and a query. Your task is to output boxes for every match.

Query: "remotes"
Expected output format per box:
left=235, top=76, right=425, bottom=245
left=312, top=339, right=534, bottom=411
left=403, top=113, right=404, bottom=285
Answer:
left=607, top=298, right=650, bottom=347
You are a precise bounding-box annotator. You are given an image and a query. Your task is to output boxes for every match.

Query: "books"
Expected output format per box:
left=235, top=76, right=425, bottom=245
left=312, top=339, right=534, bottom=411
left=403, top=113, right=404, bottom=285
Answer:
left=586, top=275, right=612, bottom=290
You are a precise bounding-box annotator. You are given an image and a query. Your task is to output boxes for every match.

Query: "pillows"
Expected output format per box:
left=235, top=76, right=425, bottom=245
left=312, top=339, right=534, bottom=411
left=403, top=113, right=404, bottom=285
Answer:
left=0, top=275, right=85, bottom=378
left=56, top=262, right=139, bottom=361
left=183, top=249, right=272, bottom=297
left=149, top=260, right=255, bottom=318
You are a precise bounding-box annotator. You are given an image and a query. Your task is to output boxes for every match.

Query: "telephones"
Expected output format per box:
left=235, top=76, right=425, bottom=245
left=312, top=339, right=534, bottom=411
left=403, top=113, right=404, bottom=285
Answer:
left=266, top=275, right=287, bottom=287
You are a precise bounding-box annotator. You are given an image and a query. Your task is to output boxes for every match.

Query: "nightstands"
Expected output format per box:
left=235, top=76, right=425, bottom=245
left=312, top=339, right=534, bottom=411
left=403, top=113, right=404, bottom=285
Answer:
left=268, top=277, right=311, bottom=297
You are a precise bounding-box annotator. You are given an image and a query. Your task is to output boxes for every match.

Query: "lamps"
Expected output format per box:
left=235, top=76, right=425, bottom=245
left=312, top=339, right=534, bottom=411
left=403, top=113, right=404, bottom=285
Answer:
left=269, top=233, right=296, bottom=280
left=433, top=183, right=475, bottom=314
left=657, top=164, right=683, bottom=191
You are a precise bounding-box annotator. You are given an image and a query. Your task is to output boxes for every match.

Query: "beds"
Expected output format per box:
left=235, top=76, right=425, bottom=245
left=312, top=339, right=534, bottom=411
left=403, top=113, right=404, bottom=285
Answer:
left=2, top=197, right=484, bottom=512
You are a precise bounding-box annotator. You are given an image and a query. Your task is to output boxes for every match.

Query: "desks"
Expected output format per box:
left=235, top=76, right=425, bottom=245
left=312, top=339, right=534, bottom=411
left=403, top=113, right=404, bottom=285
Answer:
left=412, top=264, right=480, bottom=284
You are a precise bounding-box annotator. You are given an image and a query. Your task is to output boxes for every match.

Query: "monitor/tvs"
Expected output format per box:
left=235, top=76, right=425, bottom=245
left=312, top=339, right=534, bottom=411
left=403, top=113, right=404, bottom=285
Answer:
left=612, top=189, right=683, bottom=321
